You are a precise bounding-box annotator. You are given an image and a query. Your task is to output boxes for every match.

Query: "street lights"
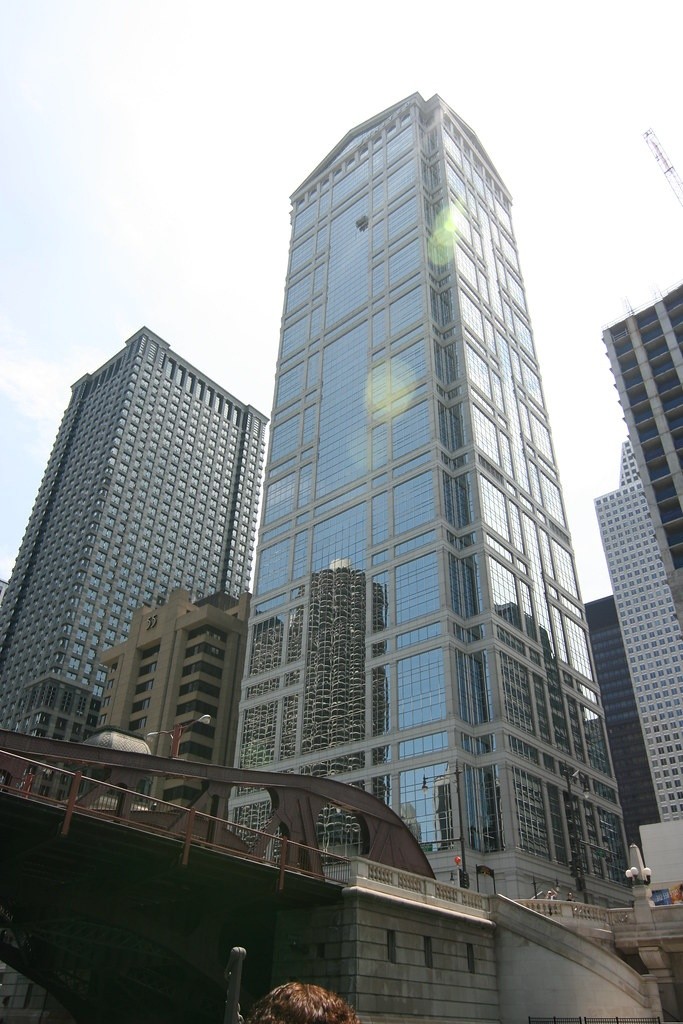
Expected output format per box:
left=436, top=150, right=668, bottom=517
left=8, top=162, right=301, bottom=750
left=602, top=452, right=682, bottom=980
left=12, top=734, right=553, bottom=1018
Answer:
left=146, top=714, right=212, bottom=758
left=529, top=876, right=560, bottom=908
left=422, top=757, right=471, bottom=888
left=562, top=762, right=592, bottom=918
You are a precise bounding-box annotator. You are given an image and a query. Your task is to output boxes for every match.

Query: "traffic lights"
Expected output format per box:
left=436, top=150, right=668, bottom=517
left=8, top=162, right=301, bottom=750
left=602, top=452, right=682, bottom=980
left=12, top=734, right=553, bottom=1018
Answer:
left=464, top=871, right=468, bottom=884
left=568, top=860, right=573, bottom=878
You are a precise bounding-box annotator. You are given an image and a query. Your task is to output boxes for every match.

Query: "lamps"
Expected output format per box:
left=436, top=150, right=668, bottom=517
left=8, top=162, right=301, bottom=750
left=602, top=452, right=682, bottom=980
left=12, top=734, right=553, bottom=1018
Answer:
left=626, top=870, right=634, bottom=884
left=644, top=868, right=652, bottom=884
left=631, top=867, right=641, bottom=882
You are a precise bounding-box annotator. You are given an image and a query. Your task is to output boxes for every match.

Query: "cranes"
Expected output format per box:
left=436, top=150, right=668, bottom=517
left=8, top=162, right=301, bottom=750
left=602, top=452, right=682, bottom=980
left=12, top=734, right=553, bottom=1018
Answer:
left=642, top=126, right=683, bottom=210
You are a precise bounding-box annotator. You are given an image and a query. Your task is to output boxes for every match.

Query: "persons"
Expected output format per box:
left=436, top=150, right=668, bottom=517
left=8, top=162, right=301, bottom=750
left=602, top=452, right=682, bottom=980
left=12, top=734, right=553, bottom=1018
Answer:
left=241, top=982, right=363, bottom=1024
